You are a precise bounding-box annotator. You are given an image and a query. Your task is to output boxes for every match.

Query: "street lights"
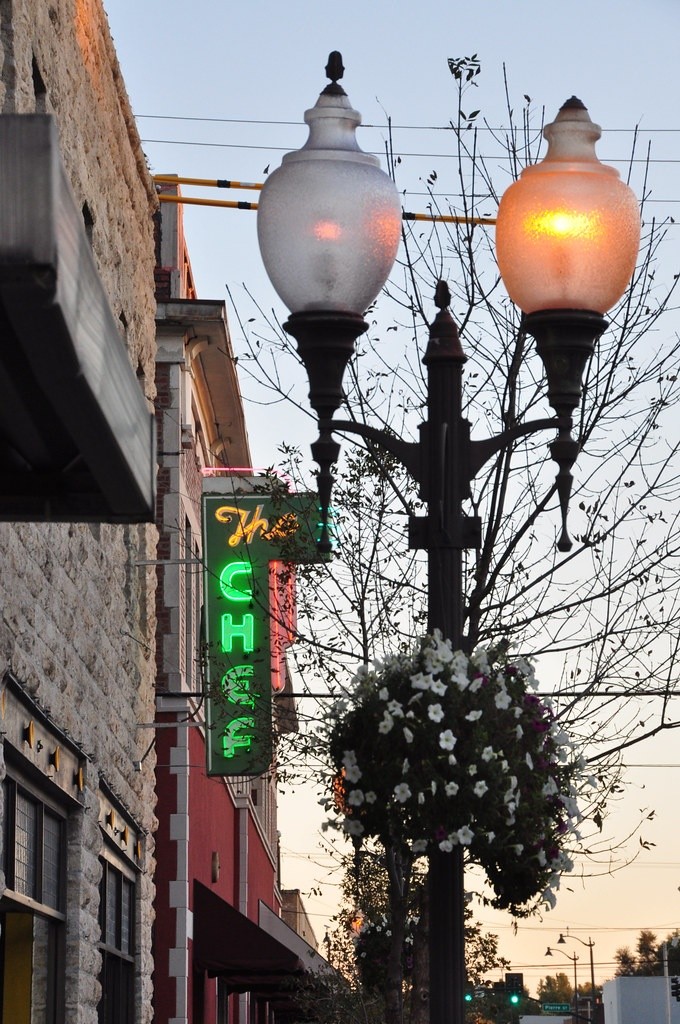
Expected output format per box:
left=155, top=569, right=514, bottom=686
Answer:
left=254, top=49, right=641, bottom=1024
left=545, top=946, right=579, bottom=1023
left=557, top=933, right=598, bottom=1023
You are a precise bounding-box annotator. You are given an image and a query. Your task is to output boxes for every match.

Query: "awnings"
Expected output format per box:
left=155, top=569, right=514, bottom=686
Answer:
left=1, top=112, right=160, bottom=528
left=193, top=878, right=351, bottom=1007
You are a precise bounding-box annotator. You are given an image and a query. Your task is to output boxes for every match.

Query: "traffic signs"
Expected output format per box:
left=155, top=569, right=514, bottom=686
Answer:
left=542, top=1003, right=571, bottom=1013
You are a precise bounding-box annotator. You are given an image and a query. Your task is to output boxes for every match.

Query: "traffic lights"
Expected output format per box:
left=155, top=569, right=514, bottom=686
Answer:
left=465, top=981, right=473, bottom=1002
left=511, top=985, right=519, bottom=1004
left=671, top=977, right=679, bottom=1002
